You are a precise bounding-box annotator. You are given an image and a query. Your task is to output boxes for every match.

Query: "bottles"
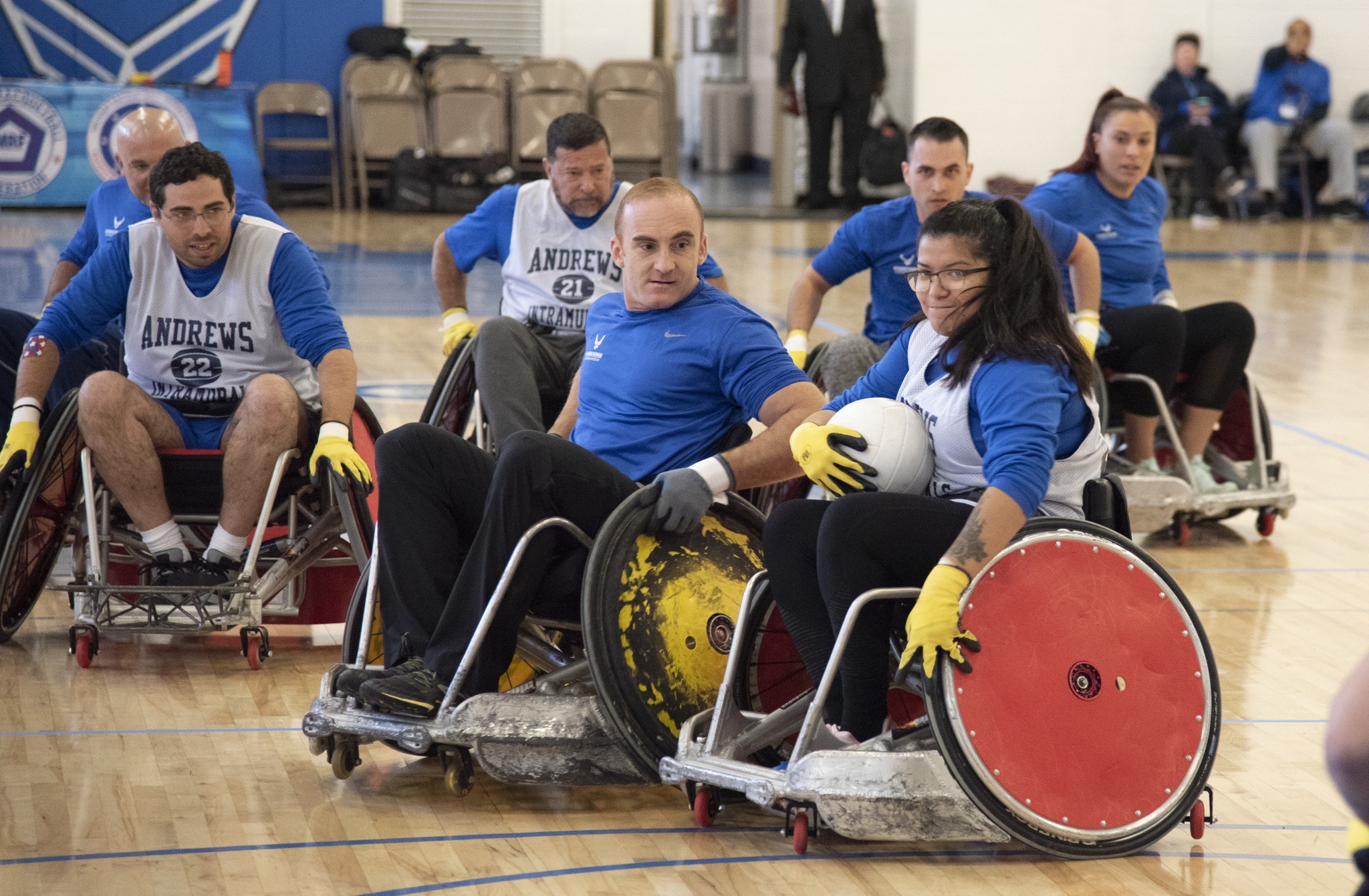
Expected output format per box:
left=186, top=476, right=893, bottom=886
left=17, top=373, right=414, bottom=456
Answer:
left=217, top=47, right=232, bottom=87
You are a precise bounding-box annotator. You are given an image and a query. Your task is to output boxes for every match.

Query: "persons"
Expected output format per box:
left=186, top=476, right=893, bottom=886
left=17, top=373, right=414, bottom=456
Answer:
left=336, top=178, right=829, bottom=716
left=431, top=113, right=729, bottom=454
left=1241, top=20, right=1360, bottom=227
left=765, top=198, right=1107, bottom=760
left=774, top=1, right=887, bottom=210
left=0, top=106, right=374, bottom=607
left=1021, top=89, right=1257, bottom=495
left=1150, top=20, right=1252, bottom=229
left=784, top=117, right=1102, bottom=399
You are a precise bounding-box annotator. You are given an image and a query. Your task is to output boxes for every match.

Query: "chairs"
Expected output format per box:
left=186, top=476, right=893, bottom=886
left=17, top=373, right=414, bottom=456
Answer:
left=255, top=53, right=675, bottom=210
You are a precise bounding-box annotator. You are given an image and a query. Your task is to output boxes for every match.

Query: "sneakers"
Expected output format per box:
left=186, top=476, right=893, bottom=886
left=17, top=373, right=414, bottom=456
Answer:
left=197, top=548, right=243, bottom=604
left=138, top=547, right=192, bottom=602
left=358, top=669, right=471, bottom=720
left=337, top=656, right=426, bottom=707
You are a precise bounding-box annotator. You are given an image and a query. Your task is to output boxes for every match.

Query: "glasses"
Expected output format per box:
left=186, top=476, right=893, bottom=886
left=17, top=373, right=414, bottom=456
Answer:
left=156, top=203, right=233, bottom=225
left=906, top=266, right=990, bottom=293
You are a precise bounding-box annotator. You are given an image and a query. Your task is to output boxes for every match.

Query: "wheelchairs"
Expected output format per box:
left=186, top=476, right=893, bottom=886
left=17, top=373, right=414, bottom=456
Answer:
left=298, top=423, right=781, bottom=801
left=658, top=520, right=1222, bottom=858
left=1091, top=359, right=1297, bottom=547
left=414, top=331, right=495, bottom=455
left=2, top=389, right=390, bottom=669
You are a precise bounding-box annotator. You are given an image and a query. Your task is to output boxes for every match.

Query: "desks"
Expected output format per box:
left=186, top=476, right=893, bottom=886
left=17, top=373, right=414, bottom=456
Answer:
left=0, top=78, right=264, bottom=207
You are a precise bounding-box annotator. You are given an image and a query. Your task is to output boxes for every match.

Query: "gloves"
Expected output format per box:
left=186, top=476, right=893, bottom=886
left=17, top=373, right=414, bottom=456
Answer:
left=893, top=563, right=981, bottom=685
left=790, top=423, right=879, bottom=498
left=308, top=420, right=373, bottom=500
left=440, top=307, right=476, bottom=356
left=641, top=452, right=736, bottom=536
left=783, top=329, right=809, bottom=370
left=1075, top=311, right=1101, bottom=363
left=0, top=396, right=42, bottom=478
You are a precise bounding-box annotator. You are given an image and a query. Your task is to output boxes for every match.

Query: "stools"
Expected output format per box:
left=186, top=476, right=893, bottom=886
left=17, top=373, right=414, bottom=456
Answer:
left=1150, top=149, right=1312, bottom=220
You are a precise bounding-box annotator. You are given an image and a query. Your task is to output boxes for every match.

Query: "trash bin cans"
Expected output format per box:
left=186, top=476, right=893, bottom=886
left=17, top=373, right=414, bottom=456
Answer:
left=699, top=75, right=753, bottom=175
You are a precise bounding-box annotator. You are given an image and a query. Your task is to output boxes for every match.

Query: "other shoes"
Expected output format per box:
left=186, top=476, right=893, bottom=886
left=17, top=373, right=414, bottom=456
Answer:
left=1187, top=198, right=1222, bottom=223
left=1134, top=456, right=1165, bottom=476
left=1178, top=454, right=1239, bottom=493
left=771, top=710, right=891, bottom=772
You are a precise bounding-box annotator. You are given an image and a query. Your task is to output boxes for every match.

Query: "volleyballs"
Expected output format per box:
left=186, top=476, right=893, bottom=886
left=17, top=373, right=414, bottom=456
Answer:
left=824, top=393, right=936, bottom=498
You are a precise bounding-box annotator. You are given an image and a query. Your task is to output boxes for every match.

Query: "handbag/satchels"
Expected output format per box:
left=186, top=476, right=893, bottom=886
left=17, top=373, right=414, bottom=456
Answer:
left=859, top=97, right=907, bottom=201
